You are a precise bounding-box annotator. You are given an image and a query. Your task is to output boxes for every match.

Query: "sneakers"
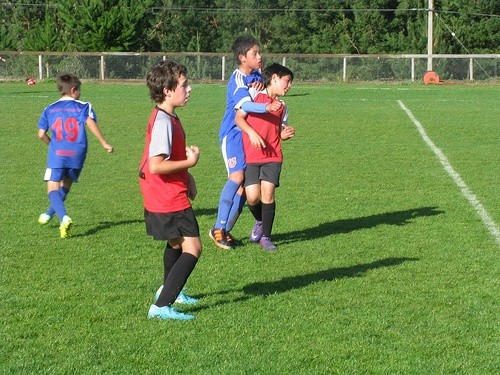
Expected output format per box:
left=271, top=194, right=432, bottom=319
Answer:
left=250, top=222, right=263, bottom=242
left=59, top=215, right=74, bottom=238
left=148, top=304, right=196, bottom=320
left=155, top=285, right=201, bottom=304
left=259, top=236, right=276, bottom=249
left=38, top=213, right=51, bottom=226
left=209, top=224, right=244, bottom=250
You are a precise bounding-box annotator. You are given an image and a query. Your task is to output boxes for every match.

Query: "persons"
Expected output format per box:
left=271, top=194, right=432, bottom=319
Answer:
left=209, top=35, right=281, bottom=250
left=38, top=74, right=113, bottom=239
left=139, top=60, right=202, bottom=321
left=234, top=62, right=295, bottom=249
